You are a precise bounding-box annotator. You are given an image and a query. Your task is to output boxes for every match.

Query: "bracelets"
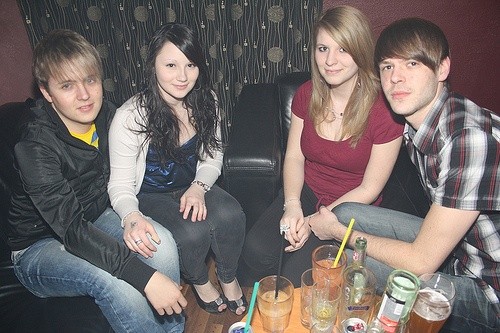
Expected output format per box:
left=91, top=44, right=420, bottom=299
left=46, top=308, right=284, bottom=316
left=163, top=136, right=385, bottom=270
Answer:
left=191, top=180, right=211, bottom=192
left=282, top=199, right=302, bottom=211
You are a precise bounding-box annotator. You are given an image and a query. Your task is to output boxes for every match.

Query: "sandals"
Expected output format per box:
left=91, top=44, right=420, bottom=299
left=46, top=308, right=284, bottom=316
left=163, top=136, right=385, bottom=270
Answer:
left=190, top=283, right=227, bottom=315
left=217, top=275, right=248, bottom=316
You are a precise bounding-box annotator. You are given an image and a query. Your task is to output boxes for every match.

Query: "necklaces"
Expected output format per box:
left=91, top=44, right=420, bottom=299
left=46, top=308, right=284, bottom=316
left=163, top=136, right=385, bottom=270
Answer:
left=323, top=104, right=346, bottom=116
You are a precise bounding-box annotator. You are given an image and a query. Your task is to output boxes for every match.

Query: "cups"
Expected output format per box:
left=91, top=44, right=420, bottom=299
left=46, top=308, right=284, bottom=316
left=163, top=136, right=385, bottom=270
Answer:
left=407, top=273, right=456, bottom=333
left=312, top=245, right=348, bottom=301
left=309, top=280, right=342, bottom=333
left=375, top=270, right=421, bottom=327
left=300, top=268, right=330, bottom=329
left=228, top=321, right=252, bottom=333
left=342, top=266, right=377, bottom=328
left=257, top=276, right=294, bottom=333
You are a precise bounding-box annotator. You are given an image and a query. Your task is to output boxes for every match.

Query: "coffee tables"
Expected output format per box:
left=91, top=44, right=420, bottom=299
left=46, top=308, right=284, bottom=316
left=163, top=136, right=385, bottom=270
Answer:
left=233, top=284, right=443, bottom=333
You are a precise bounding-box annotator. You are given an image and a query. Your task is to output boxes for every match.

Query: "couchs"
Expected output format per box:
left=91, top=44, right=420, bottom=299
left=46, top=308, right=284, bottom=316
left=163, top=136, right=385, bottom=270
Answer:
left=225, top=68, right=333, bottom=225
left=0, top=95, right=115, bottom=333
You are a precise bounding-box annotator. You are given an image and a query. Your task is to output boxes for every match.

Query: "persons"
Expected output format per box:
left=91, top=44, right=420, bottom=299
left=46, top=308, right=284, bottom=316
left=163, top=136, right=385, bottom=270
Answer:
left=6, top=28, right=187, bottom=333
left=106, top=22, right=249, bottom=316
left=242, top=6, right=405, bottom=289
left=308, top=17, right=500, bottom=333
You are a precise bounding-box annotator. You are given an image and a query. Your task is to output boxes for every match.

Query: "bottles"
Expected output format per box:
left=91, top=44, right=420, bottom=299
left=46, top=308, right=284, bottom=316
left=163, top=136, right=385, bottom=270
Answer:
left=341, top=317, right=368, bottom=333
left=342, top=236, right=368, bottom=305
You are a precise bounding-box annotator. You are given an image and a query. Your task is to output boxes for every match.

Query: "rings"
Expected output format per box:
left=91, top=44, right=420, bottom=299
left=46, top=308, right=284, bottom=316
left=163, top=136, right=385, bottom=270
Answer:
left=280, top=224, right=291, bottom=232
left=134, top=239, right=142, bottom=245
